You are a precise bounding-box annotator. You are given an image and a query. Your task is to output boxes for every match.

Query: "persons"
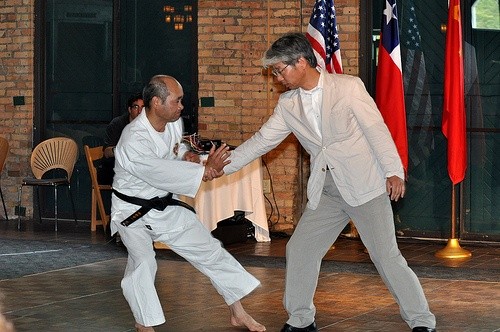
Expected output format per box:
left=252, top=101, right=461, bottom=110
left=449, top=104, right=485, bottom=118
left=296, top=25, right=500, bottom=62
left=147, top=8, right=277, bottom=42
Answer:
left=99, top=91, right=144, bottom=185
left=110, top=75, right=266, bottom=332
left=204, top=33, right=437, bottom=331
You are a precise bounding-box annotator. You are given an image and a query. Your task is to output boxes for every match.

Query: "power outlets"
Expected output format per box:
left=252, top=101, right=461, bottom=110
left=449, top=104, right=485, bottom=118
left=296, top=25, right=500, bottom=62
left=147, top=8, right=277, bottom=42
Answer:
left=263, top=180, right=270, bottom=192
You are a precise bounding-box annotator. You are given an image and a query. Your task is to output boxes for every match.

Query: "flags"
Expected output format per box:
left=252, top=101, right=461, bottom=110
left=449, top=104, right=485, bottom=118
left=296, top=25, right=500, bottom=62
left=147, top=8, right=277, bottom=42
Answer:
left=442, top=0, right=468, bottom=184
left=306, top=0, right=343, bottom=73
left=376, top=0, right=408, bottom=182
left=464, top=21, right=486, bottom=161
left=400, top=0, right=435, bottom=152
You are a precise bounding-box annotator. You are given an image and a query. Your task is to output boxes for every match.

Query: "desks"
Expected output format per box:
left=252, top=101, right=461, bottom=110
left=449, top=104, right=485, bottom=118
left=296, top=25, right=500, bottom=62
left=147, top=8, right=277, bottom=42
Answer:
left=154, top=151, right=272, bottom=249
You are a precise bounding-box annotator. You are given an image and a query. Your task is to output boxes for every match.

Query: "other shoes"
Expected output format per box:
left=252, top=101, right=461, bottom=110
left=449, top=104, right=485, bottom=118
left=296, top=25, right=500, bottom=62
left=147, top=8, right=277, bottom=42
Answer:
left=280, top=321, right=317, bottom=332
left=412, top=327, right=436, bottom=332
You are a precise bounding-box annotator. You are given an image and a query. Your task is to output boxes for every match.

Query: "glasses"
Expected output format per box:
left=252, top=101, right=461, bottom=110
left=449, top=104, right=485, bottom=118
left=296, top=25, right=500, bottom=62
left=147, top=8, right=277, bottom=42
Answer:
left=131, top=105, right=143, bottom=110
left=272, top=64, right=289, bottom=76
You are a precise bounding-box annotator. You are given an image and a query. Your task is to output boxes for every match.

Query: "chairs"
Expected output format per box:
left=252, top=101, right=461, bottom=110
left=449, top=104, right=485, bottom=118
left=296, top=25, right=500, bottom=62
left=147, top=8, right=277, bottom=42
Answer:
left=0, top=136, right=10, bottom=222
left=86, top=144, right=110, bottom=233
left=17, top=137, right=80, bottom=231
